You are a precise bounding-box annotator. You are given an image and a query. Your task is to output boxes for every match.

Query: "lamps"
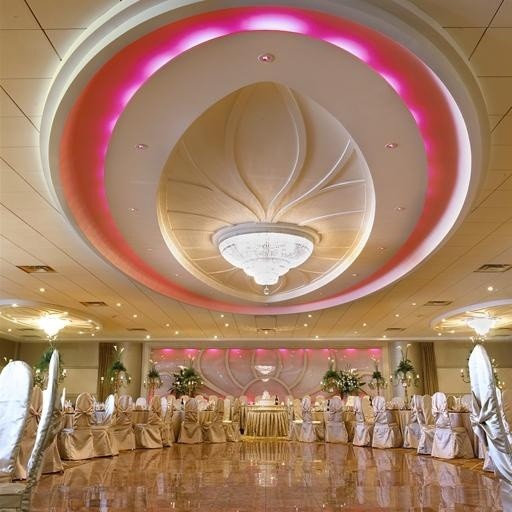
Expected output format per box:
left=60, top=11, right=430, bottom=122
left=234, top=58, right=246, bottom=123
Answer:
left=209, top=221, right=321, bottom=295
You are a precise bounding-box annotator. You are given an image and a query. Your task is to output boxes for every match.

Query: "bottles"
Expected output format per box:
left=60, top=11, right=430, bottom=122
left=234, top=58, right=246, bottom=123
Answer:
left=369, top=396, right=372, bottom=406
left=180, top=398, right=184, bottom=410
left=326, top=400, right=330, bottom=411
left=275, top=395, right=278, bottom=406
